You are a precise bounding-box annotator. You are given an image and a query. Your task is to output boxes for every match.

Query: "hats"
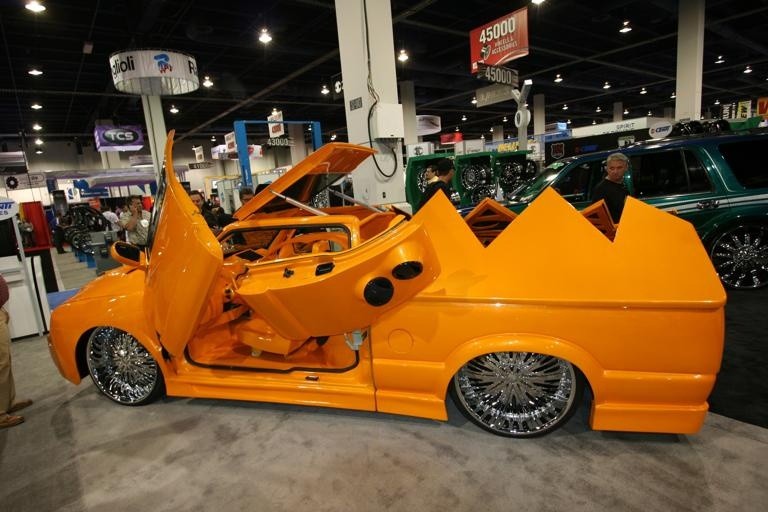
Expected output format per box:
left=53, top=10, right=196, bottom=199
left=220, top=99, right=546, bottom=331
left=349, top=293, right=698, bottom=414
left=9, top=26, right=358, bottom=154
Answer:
left=438, top=159, right=456, bottom=170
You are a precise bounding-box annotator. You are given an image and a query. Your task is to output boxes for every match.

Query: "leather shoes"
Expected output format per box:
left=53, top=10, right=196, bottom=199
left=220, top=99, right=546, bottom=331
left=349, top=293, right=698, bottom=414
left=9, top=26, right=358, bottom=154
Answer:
left=0, top=415, right=25, bottom=429
left=8, top=398, right=33, bottom=413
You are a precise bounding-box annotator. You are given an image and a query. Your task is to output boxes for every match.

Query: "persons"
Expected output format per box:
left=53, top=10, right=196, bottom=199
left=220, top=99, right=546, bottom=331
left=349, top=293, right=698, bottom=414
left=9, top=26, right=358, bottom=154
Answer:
left=0, top=269, right=32, bottom=431
left=592, top=152, right=633, bottom=226
left=416, top=159, right=457, bottom=210
left=425, top=167, right=437, bottom=185
left=99, top=187, right=257, bottom=261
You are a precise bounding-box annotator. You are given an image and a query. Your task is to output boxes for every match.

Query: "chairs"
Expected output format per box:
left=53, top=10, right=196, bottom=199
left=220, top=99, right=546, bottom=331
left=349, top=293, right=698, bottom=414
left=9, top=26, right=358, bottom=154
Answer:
left=235, top=240, right=334, bottom=355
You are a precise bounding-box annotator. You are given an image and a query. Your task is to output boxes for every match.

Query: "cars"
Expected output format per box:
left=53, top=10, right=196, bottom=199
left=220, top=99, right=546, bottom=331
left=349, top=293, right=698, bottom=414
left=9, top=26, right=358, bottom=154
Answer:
left=455, top=127, right=768, bottom=292
left=47, top=128, right=727, bottom=438
